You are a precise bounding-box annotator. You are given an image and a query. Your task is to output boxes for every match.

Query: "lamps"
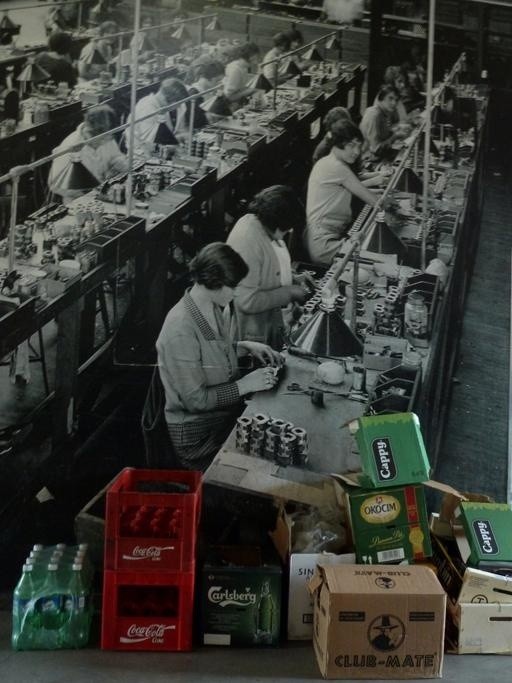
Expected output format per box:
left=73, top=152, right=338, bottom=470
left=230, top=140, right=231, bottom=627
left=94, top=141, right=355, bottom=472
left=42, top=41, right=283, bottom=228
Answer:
left=436, top=68, right=473, bottom=108
left=292, top=132, right=441, bottom=357
left=15, top=55, right=53, bottom=81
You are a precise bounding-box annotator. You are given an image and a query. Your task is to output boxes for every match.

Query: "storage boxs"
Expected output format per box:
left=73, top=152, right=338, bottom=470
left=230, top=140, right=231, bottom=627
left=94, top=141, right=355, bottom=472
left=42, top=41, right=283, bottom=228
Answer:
left=101, top=466, right=204, bottom=650
left=199, top=533, right=282, bottom=647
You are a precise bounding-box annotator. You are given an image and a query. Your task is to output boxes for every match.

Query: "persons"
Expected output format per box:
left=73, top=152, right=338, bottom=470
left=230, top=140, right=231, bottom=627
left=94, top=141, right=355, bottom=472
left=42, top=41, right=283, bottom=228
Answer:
left=153, top=240, right=287, bottom=517
left=225, top=184, right=318, bottom=354
left=1, top=1, right=427, bottom=268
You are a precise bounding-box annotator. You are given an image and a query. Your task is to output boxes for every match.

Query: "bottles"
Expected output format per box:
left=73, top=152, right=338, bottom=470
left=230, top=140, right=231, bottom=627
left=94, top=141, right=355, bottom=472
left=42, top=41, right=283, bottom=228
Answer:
left=210, top=38, right=240, bottom=51
left=317, top=350, right=421, bottom=392
left=31, top=100, right=48, bottom=124
left=96, top=146, right=172, bottom=208
left=10, top=199, right=106, bottom=265
left=461, top=83, right=481, bottom=101
left=38, top=80, right=68, bottom=99
left=254, top=580, right=276, bottom=643
left=236, top=414, right=310, bottom=468
left=13, top=544, right=93, bottom=650
left=404, top=293, right=427, bottom=335
left=121, top=64, right=129, bottom=82
left=319, top=61, right=332, bottom=74
left=1, top=119, right=16, bottom=137
left=18, top=271, right=49, bottom=309
left=481, top=70, right=487, bottom=78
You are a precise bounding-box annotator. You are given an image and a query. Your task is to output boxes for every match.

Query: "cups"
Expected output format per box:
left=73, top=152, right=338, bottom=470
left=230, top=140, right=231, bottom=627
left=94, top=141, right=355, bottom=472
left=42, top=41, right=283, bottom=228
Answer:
left=142, top=52, right=166, bottom=81
left=189, top=135, right=214, bottom=158
